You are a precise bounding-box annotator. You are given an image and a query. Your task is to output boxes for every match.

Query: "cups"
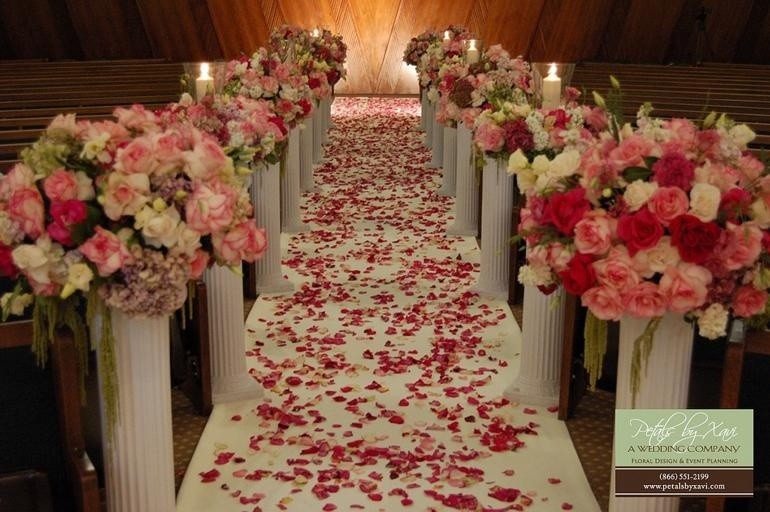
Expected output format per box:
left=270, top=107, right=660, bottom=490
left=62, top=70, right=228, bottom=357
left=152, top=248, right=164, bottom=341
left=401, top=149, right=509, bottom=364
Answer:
left=531, top=63, right=575, bottom=112
left=440, top=31, right=456, bottom=45
left=302, top=24, right=329, bottom=42
left=462, top=39, right=484, bottom=67
left=184, top=61, right=225, bottom=102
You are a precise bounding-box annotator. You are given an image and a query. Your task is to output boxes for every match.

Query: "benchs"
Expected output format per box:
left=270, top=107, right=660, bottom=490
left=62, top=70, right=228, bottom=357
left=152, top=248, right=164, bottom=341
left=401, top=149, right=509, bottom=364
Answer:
left=399, top=22, right=769, bottom=410
left=1, top=18, right=349, bottom=486
